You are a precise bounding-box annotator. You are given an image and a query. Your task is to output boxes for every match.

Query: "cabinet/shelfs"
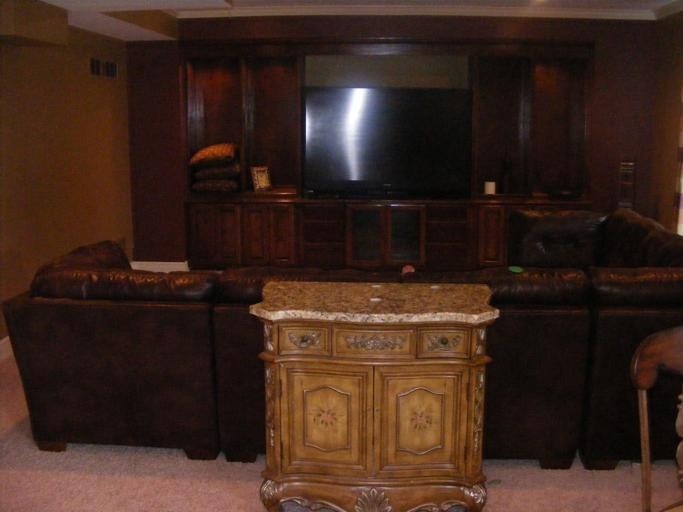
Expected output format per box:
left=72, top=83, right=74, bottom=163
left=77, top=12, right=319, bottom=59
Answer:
left=476, top=203, right=504, bottom=267
left=188, top=203, right=240, bottom=268
left=241, top=201, right=294, bottom=271
left=388, top=202, right=473, bottom=267
left=298, top=201, right=386, bottom=271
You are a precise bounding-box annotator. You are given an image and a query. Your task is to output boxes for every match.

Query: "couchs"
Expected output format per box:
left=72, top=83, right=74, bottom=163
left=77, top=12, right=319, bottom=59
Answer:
left=6, top=240, right=217, bottom=459
left=508, top=202, right=682, bottom=470
left=217, top=264, right=591, bottom=467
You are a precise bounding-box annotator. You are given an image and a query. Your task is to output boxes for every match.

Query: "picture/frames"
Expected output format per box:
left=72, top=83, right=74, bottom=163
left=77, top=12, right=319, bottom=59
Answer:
left=251, top=166, right=272, bottom=193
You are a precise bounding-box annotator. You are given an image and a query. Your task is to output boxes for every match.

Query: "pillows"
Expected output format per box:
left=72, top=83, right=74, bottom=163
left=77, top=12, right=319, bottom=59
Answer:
left=187, top=142, right=241, bottom=195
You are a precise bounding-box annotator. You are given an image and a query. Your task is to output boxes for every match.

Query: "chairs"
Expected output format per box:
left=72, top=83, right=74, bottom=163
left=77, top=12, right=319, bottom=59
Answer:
left=630, top=325, right=683, bottom=512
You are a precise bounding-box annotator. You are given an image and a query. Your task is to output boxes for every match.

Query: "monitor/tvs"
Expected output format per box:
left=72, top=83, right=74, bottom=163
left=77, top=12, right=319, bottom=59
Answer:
left=301, top=85, right=473, bottom=200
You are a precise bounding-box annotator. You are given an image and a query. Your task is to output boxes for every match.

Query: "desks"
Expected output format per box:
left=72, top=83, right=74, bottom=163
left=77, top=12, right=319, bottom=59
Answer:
left=248, top=280, right=499, bottom=512
